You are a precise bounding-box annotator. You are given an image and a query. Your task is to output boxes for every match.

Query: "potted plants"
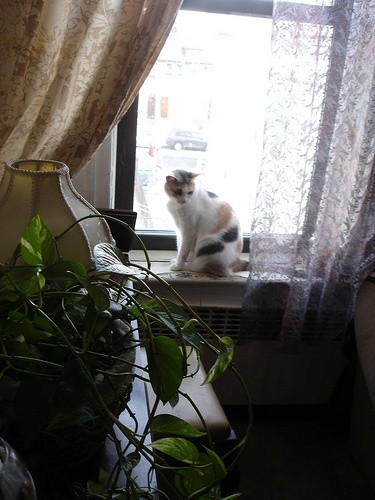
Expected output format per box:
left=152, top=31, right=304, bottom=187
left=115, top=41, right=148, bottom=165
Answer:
left=0, top=214, right=252, bottom=500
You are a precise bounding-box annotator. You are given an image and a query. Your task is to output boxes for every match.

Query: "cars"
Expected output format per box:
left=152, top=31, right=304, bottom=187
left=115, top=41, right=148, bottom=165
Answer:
left=164, top=130, right=206, bottom=150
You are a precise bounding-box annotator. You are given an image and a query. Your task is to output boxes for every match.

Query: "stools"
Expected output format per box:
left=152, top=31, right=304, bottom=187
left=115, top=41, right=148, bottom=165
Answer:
left=141, top=345, right=238, bottom=448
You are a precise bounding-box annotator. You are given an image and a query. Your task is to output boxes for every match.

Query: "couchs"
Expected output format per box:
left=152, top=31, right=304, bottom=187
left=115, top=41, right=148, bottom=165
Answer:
left=349, top=266, right=375, bottom=493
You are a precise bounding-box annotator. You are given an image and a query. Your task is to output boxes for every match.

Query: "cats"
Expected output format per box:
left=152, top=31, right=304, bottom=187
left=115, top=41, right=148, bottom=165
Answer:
left=164, top=169, right=321, bottom=280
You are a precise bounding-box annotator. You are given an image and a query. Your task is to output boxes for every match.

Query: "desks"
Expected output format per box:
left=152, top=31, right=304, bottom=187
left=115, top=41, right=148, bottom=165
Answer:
left=19, top=278, right=156, bottom=499
